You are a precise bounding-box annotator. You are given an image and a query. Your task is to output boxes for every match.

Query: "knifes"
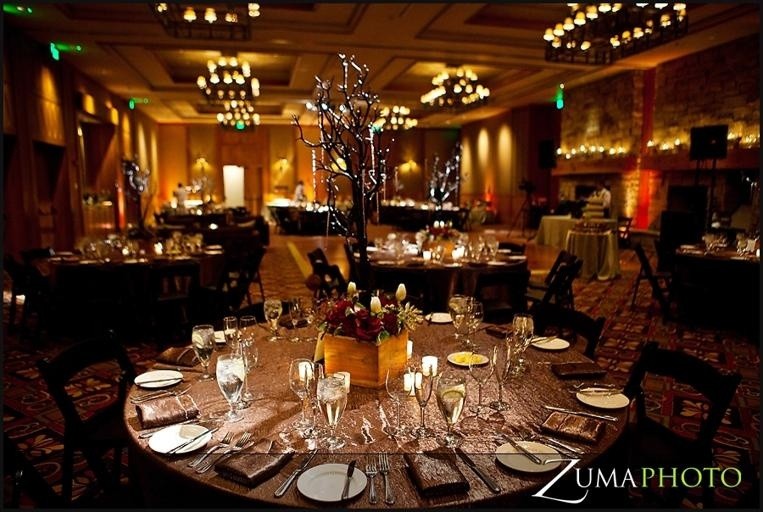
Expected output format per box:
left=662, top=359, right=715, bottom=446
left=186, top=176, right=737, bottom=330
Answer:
left=541, top=404, right=620, bottom=424
left=340, top=458, right=358, bottom=500
left=499, top=431, right=539, bottom=465
left=454, top=446, right=502, bottom=494
left=273, top=449, right=317, bottom=499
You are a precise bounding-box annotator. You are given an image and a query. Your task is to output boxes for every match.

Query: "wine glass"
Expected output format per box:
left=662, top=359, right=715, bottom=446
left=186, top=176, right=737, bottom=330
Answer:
left=192, top=297, right=317, bottom=424
left=288, top=359, right=348, bottom=450
left=386, top=292, right=535, bottom=438
left=370, top=232, right=501, bottom=265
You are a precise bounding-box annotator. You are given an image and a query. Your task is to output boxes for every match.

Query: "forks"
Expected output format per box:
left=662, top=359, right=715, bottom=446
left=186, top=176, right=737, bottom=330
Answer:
left=187, top=432, right=251, bottom=474
left=365, top=452, right=396, bottom=505
left=130, top=384, right=195, bottom=404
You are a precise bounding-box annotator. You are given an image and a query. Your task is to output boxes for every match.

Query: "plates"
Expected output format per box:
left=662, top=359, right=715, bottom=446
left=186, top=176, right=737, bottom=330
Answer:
left=298, top=464, right=368, bottom=501
left=134, top=370, right=186, bottom=387
left=495, top=443, right=563, bottom=473
left=574, top=387, right=630, bottom=409
left=532, top=335, right=570, bottom=351
left=149, top=424, right=213, bottom=455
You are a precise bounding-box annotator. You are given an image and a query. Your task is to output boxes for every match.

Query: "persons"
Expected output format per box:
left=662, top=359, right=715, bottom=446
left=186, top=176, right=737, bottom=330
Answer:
left=294, top=180, right=305, bottom=201
left=173, top=182, right=186, bottom=208
left=580, top=178, right=611, bottom=218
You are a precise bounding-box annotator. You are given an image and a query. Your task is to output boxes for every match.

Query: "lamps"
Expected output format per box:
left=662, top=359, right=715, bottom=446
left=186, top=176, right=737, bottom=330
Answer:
left=544, top=0, right=691, bottom=66
left=421, top=62, right=490, bottom=114
left=150, top=0, right=252, bottom=43
left=196, top=51, right=261, bottom=100
left=216, top=99, right=260, bottom=130
